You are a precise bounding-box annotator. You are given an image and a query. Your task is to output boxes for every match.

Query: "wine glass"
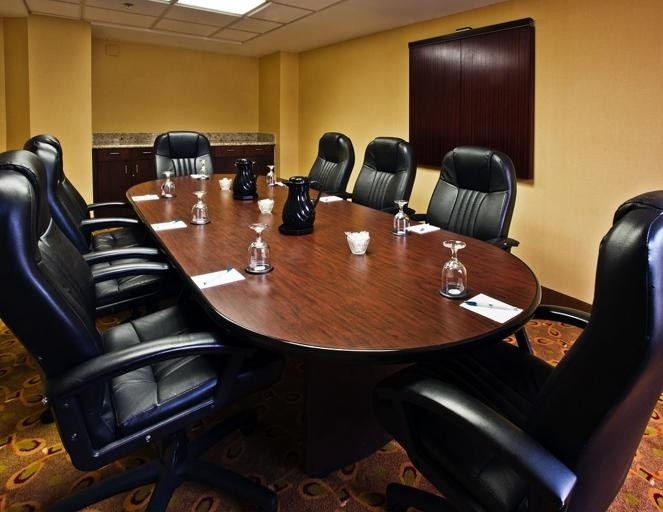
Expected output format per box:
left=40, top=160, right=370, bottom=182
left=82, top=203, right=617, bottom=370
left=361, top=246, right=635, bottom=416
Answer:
left=265, top=164, right=277, bottom=186
left=248, top=223, right=272, bottom=273
left=440, top=239, right=468, bottom=299
left=392, top=200, right=410, bottom=236
left=190, top=191, right=209, bottom=224
left=161, top=170, right=175, bottom=198
left=199, top=159, right=208, bottom=178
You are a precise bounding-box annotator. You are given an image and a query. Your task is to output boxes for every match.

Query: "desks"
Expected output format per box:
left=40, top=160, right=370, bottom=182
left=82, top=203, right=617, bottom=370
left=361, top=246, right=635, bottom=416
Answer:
left=125, top=173, right=544, bottom=478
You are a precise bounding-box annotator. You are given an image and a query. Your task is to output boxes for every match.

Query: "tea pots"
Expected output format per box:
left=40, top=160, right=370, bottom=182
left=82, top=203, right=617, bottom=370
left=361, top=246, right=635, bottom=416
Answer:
left=232, top=158, right=259, bottom=195
left=282, top=176, right=323, bottom=230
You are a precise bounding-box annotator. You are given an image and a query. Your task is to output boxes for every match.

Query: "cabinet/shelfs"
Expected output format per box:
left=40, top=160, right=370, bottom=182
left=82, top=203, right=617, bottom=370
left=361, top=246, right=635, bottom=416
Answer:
left=93, top=145, right=274, bottom=228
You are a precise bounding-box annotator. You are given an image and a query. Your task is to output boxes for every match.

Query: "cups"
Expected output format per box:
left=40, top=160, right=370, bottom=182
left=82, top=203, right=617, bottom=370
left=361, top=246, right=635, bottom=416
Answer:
left=347, top=236, right=370, bottom=255
left=257, top=200, right=275, bottom=214
left=218, top=179, right=233, bottom=191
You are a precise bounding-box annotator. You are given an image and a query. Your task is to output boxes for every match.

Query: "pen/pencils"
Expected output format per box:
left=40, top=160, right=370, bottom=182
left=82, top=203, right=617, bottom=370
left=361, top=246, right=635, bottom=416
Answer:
left=170, top=219, right=178, bottom=225
left=203, top=265, right=234, bottom=285
left=419, top=222, right=431, bottom=232
left=465, top=301, right=518, bottom=310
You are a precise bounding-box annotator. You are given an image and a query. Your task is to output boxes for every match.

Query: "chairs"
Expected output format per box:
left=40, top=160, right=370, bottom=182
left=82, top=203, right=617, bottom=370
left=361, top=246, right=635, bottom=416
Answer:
left=405, top=147, right=533, bottom=358
left=2, top=149, right=280, bottom=512
left=384, top=189, right=663, bottom=512
left=150, top=132, right=215, bottom=178
left=303, top=133, right=356, bottom=197
left=338, top=136, right=418, bottom=215
left=24, top=134, right=183, bottom=312
